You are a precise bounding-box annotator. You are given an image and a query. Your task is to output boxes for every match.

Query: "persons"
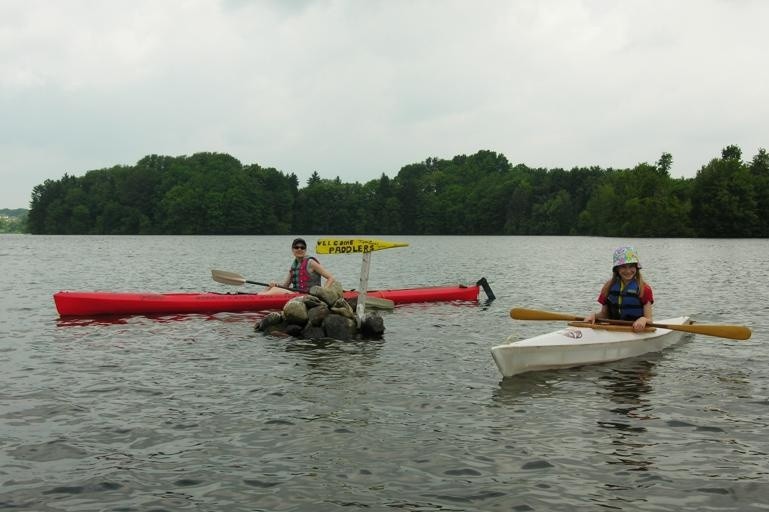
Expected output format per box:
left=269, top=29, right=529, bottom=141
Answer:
left=257, top=238, right=336, bottom=295
left=584, top=246, right=654, bottom=329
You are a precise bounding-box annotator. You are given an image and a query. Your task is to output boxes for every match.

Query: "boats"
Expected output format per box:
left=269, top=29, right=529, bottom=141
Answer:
left=53, top=286, right=481, bottom=317
left=490, top=316, right=692, bottom=378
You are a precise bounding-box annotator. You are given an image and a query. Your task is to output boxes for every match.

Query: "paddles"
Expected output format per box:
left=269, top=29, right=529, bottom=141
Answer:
left=212, top=270, right=394, bottom=310
left=507, top=308, right=751, bottom=340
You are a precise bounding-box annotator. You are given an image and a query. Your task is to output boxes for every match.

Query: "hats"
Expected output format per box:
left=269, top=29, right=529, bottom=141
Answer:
left=292, top=238, right=306, bottom=247
left=611, top=245, right=643, bottom=269
